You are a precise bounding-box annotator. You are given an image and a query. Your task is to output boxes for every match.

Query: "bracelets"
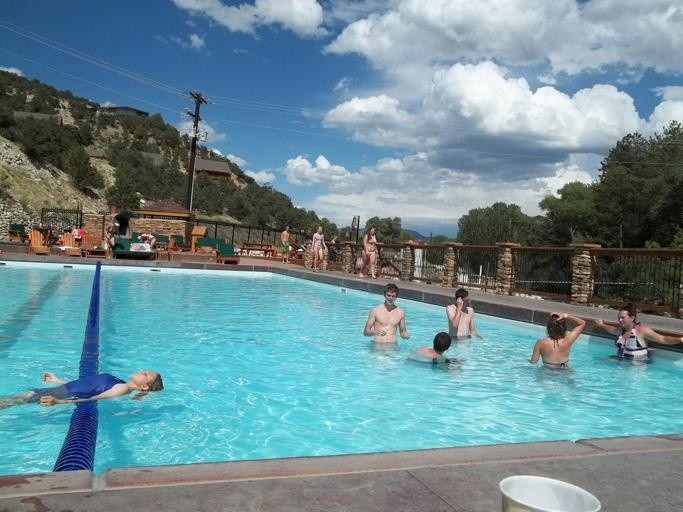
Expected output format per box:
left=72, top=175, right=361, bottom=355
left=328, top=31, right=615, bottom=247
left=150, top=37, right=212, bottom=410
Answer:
left=565, top=313, right=568, bottom=320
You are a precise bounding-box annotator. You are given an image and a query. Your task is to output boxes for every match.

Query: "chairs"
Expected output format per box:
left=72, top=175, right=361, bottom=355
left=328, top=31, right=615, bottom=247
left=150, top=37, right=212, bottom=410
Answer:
left=8, top=223, right=27, bottom=243
left=27, top=229, right=51, bottom=256
left=55, top=231, right=108, bottom=258
left=155, top=234, right=183, bottom=251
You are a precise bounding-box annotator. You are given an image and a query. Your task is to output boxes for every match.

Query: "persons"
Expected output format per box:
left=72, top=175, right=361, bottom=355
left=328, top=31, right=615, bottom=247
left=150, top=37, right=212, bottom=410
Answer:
left=137, top=234, right=156, bottom=250
left=280, top=226, right=291, bottom=263
left=310, top=225, right=327, bottom=273
left=0, top=371, right=165, bottom=407
left=364, top=283, right=410, bottom=345
left=530, top=312, right=586, bottom=369
left=417, top=332, right=451, bottom=357
left=593, top=302, right=683, bottom=361
left=103, top=222, right=115, bottom=259
left=330, top=235, right=338, bottom=263
left=359, top=225, right=378, bottom=279
left=446, top=289, right=477, bottom=341
left=47, top=222, right=85, bottom=248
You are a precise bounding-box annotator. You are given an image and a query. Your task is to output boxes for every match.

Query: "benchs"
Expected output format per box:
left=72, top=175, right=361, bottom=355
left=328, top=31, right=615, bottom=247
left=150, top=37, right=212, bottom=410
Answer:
left=112, top=238, right=158, bottom=260
left=240, top=242, right=275, bottom=256
left=197, top=237, right=241, bottom=265
